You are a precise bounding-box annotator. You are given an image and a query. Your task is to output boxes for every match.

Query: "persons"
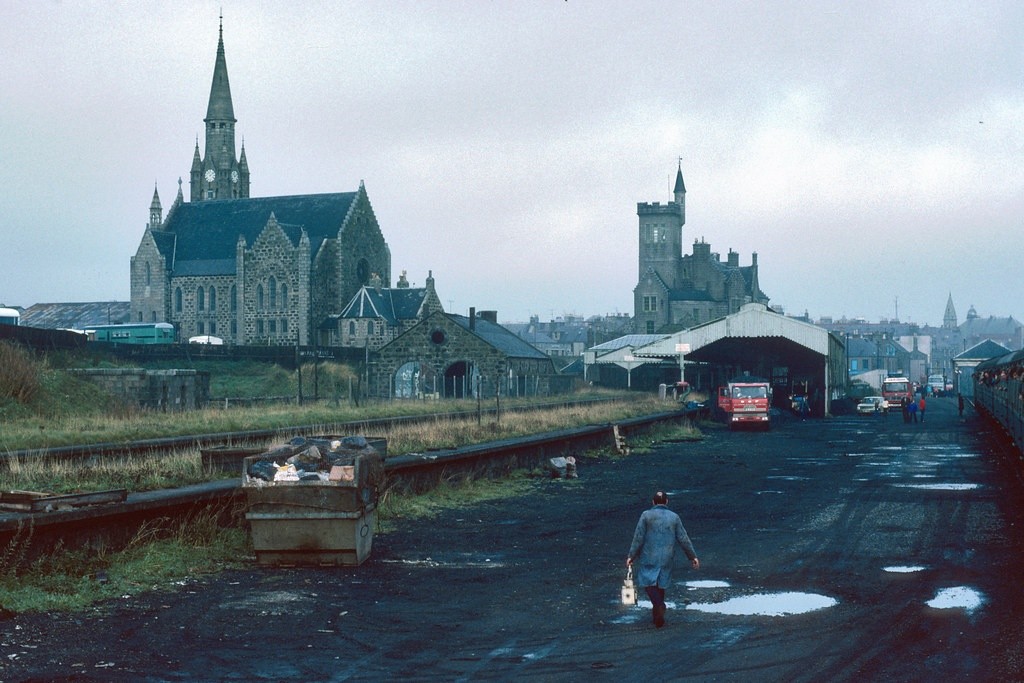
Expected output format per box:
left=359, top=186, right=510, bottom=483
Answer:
left=626, top=491, right=699, bottom=629
left=901, top=394, right=918, bottom=424
left=958, top=393, right=964, bottom=417
left=882, top=399, right=889, bottom=415
left=971, top=366, right=1024, bottom=401
left=799, top=394, right=811, bottom=422
left=919, top=396, right=926, bottom=422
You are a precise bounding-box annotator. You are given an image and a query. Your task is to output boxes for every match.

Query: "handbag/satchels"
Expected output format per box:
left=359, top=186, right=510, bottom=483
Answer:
left=621, top=565, right=637, bottom=606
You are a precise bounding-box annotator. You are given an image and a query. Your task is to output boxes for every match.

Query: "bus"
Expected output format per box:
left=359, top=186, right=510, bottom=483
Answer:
left=83, top=322, right=174, bottom=343
left=0, top=307, right=20, bottom=327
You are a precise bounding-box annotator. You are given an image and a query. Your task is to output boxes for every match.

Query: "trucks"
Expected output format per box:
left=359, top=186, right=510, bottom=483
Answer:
left=717, top=380, right=774, bottom=432
left=927, top=376, right=945, bottom=391
left=881, top=377, right=916, bottom=409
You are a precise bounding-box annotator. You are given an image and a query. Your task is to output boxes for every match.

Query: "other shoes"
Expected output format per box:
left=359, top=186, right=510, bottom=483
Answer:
left=653, top=603, right=666, bottom=629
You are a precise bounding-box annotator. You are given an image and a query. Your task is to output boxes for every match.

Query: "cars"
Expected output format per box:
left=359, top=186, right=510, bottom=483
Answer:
left=856, top=396, right=886, bottom=413
